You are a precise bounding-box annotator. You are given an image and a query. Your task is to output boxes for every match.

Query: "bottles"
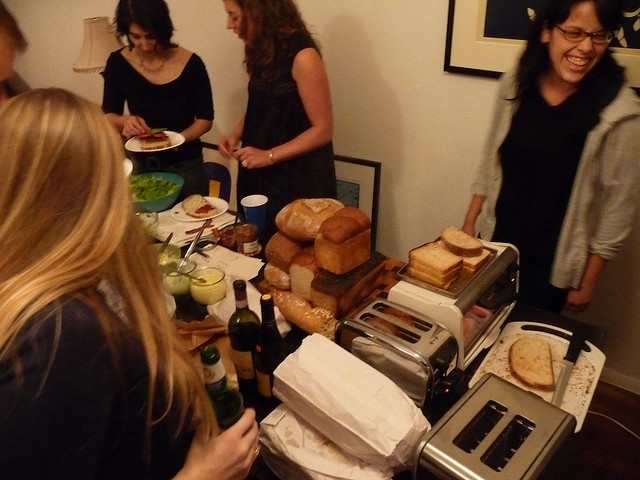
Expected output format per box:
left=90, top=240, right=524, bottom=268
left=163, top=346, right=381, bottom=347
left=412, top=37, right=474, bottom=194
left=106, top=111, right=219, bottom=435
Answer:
left=252, top=293, right=289, bottom=402
left=200, top=345, right=244, bottom=433
left=228, top=278, right=260, bottom=400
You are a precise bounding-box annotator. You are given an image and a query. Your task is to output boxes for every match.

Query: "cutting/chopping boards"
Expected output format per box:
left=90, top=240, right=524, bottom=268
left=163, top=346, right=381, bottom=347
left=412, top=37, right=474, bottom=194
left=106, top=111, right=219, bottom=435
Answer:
left=468, top=320, right=607, bottom=434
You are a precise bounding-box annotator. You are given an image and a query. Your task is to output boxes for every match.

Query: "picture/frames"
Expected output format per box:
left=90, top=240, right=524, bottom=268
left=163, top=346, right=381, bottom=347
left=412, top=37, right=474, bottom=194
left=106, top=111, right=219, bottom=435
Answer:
left=200, top=141, right=242, bottom=213
left=443, top=0, right=640, bottom=97
left=333, top=154, right=382, bottom=251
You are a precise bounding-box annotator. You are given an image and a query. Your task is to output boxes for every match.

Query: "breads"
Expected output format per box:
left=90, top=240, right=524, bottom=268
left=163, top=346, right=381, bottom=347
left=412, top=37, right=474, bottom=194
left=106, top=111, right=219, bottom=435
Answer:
left=275, top=198, right=345, bottom=241
left=264, top=232, right=304, bottom=273
left=266, top=287, right=340, bottom=342
left=406, top=226, right=491, bottom=291
left=382, top=308, right=416, bottom=328
left=508, top=337, right=555, bottom=391
left=289, top=244, right=322, bottom=302
left=138, top=129, right=171, bottom=150
left=263, top=263, right=290, bottom=290
left=183, top=193, right=216, bottom=217
left=365, top=315, right=397, bottom=336
left=314, top=206, right=371, bottom=275
left=311, top=251, right=387, bottom=319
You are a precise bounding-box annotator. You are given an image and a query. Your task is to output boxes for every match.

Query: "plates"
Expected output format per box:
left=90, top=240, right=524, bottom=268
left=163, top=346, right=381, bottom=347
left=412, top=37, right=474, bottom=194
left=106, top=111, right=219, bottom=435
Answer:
left=170, top=196, right=230, bottom=223
left=125, top=130, right=185, bottom=153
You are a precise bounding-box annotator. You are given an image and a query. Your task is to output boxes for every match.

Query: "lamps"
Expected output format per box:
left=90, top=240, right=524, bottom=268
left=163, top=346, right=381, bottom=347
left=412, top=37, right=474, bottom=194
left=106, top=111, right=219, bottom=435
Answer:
left=73, top=17, right=127, bottom=84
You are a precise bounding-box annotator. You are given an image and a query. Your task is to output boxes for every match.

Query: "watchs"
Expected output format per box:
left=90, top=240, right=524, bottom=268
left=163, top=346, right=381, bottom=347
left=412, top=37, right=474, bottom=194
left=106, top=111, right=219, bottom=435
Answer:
left=268, top=148, right=275, bottom=164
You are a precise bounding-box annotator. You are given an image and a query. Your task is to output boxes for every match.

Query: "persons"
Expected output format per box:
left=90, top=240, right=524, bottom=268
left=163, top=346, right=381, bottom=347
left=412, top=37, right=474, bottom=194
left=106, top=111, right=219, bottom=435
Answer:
left=0, top=87, right=262, bottom=479
left=218, top=0, right=337, bottom=228
left=0, top=8, right=26, bottom=104
left=458, top=2, right=640, bottom=326
left=102, top=0, right=214, bottom=183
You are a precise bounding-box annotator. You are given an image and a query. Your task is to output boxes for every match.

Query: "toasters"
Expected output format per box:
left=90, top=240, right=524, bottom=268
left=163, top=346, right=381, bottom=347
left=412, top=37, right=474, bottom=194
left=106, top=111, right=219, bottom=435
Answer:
left=333, top=295, right=459, bottom=413
left=414, top=373, right=577, bottom=480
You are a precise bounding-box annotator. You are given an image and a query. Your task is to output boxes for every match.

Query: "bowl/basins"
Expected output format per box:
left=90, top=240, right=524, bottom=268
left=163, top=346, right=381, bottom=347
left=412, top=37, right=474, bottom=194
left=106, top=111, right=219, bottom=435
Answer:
left=136, top=209, right=161, bottom=236
left=151, top=242, right=183, bottom=272
left=128, top=172, right=184, bottom=213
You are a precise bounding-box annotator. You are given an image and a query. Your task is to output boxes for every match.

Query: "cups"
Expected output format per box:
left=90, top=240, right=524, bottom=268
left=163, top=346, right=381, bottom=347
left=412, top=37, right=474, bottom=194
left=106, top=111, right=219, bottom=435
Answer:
left=161, top=259, right=197, bottom=296
left=189, top=266, right=227, bottom=305
left=238, top=223, right=259, bottom=255
left=241, top=195, right=268, bottom=228
left=221, top=226, right=237, bottom=251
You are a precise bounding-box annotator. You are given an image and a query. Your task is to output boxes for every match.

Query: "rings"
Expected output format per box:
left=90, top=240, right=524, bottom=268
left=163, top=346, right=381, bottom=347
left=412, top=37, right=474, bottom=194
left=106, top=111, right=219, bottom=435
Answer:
left=254, top=447, right=260, bottom=457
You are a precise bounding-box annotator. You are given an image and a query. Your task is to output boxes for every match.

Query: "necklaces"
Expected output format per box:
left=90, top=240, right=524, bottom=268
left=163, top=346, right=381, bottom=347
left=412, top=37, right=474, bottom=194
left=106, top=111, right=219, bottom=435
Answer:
left=133, top=44, right=168, bottom=75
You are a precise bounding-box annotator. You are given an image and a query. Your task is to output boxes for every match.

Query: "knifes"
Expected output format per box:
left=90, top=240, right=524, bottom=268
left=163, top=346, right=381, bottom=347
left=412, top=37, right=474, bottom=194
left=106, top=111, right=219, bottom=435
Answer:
left=550, top=321, right=589, bottom=408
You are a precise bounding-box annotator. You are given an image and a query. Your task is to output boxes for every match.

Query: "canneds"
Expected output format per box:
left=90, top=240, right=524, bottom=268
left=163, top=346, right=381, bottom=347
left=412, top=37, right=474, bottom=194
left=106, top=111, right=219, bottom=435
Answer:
left=235, top=224, right=257, bottom=255
left=221, top=226, right=234, bottom=247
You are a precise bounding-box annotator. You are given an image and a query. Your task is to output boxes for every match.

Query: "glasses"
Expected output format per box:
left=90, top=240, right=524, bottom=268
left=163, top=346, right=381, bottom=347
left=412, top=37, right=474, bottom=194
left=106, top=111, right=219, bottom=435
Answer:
left=555, top=25, right=614, bottom=46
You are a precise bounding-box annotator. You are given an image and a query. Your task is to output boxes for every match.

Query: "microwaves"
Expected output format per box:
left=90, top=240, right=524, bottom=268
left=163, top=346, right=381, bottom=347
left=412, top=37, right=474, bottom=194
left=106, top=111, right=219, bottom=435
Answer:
left=387, top=232, right=520, bottom=371
